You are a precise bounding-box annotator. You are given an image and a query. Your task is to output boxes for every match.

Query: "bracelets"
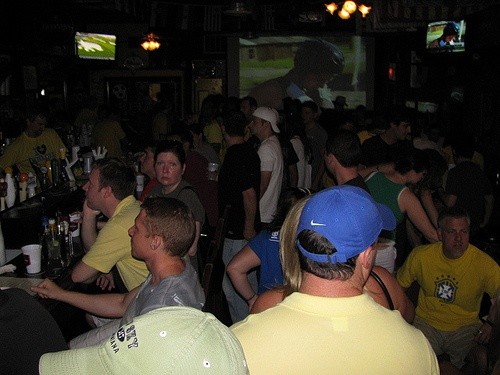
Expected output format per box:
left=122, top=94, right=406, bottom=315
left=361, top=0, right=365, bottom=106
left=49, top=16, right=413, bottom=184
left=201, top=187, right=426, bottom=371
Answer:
left=487, top=320, right=494, bottom=327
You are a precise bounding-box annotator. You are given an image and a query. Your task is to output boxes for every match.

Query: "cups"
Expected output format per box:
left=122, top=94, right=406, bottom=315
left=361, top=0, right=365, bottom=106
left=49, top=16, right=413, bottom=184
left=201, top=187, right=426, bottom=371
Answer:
left=207, top=162, right=219, bottom=180
left=135, top=175, right=144, bottom=192
left=68, top=214, right=80, bottom=238
left=21, top=244, right=42, bottom=274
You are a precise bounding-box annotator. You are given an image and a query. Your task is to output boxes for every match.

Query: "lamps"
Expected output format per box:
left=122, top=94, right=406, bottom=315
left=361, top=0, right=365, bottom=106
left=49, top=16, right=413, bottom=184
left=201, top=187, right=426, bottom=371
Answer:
left=325, top=0, right=371, bottom=19
left=141, top=33, right=160, bottom=51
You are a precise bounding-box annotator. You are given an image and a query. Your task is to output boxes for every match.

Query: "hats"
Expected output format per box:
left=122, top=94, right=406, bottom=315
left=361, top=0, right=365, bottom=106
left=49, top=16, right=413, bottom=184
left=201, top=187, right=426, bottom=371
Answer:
left=252, top=106, right=280, bottom=133
left=39, top=305, right=250, bottom=375
left=294, top=185, right=397, bottom=264
left=332, top=96, right=346, bottom=105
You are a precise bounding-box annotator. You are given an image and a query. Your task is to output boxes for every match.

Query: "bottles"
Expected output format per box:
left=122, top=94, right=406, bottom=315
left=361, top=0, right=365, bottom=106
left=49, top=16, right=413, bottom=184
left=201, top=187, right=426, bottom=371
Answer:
left=0, top=123, right=95, bottom=211
left=39, top=207, right=74, bottom=276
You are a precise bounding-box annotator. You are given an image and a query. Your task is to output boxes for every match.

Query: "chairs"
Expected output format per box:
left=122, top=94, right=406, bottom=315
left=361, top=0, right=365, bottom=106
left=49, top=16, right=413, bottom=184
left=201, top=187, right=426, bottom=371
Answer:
left=194, top=205, right=232, bottom=324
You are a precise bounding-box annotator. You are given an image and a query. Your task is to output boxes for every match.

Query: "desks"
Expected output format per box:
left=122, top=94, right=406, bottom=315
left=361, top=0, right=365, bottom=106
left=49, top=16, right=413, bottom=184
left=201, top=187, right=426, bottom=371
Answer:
left=0, top=177, right=110, bottom=342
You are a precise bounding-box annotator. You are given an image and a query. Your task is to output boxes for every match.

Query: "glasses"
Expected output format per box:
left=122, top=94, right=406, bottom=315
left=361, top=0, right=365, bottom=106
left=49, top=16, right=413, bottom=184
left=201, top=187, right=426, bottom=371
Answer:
left=156, top=161, right=177, bottom=168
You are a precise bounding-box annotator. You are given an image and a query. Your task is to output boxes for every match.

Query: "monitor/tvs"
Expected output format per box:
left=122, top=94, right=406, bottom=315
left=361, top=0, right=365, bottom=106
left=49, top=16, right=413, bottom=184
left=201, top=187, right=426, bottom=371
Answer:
left=423, top=17, right=472, bottom=57
left=73, top=30, right=118, bottom=64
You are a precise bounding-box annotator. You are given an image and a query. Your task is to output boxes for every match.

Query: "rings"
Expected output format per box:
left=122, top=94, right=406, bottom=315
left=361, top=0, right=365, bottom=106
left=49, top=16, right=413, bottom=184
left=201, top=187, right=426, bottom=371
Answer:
left=480, top=331, right=482, bottom=333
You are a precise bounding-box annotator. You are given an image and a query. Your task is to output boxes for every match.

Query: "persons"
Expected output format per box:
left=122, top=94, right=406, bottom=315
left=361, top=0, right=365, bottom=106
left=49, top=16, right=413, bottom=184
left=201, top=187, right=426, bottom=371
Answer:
left=0, top=96, right=500, bottom=375
left=228, top=185, right=441, bottom=375
left=431, top=23, right=458, bottom=46
left=395, top=206, right=500, bottom=375
left=247, top=38, right=345, bottom=109
